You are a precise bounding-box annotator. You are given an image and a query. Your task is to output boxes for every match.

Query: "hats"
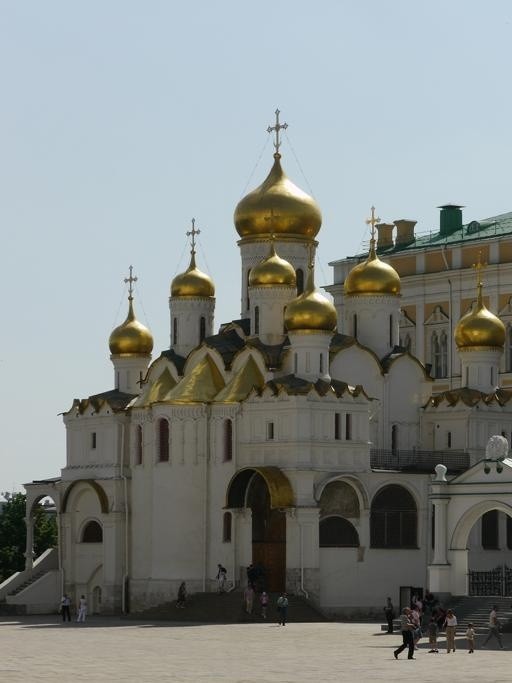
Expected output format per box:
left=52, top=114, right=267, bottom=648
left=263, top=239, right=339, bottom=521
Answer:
left=403, top=607, right=413, bottom=613
left=448, top=609, right=453, bottom=613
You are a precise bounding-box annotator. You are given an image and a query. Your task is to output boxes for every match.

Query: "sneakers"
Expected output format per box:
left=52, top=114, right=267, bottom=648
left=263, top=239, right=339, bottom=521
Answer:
left=453, top=648, right=455, bottom=652
left=469, top=650, right=473, bottom=653
left=408, top=656, right=416, bottom=659
left=429, top=650, right=438, bottom=652
left=394, top=651, right=398, bottom=659
left=448, top=650, right=450, bottom=653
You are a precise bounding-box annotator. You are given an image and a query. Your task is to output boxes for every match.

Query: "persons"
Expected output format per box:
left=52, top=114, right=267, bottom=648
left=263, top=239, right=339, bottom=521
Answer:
left=256, top=564, right=265, bottom=593
left=427, top=617, right=439, bottom=653
left=243, top=584, right=255, bottom=615
left=76, top=594, right=87, bottom=622
left=383, top=596, right=394, bottom=634
left=464, top=622, right=475, bottom=653
left=175, top=581, right=187, bottom=608
left=442, top=607, right=457, bottom=654
left=277, top=592, right=289, bottom=626
left=246, top=563, right=257, bottom=593
left=411, top=588, right=448, bottom=650
left=392, top=606, right=416, bottom=660
left=61, top=593, right=73, bottom=622
left=480, top=604, right=507, bottom=650
left=215, top=562, right=228, bottom=594
left=259, top=591, right=270, bottom=618
